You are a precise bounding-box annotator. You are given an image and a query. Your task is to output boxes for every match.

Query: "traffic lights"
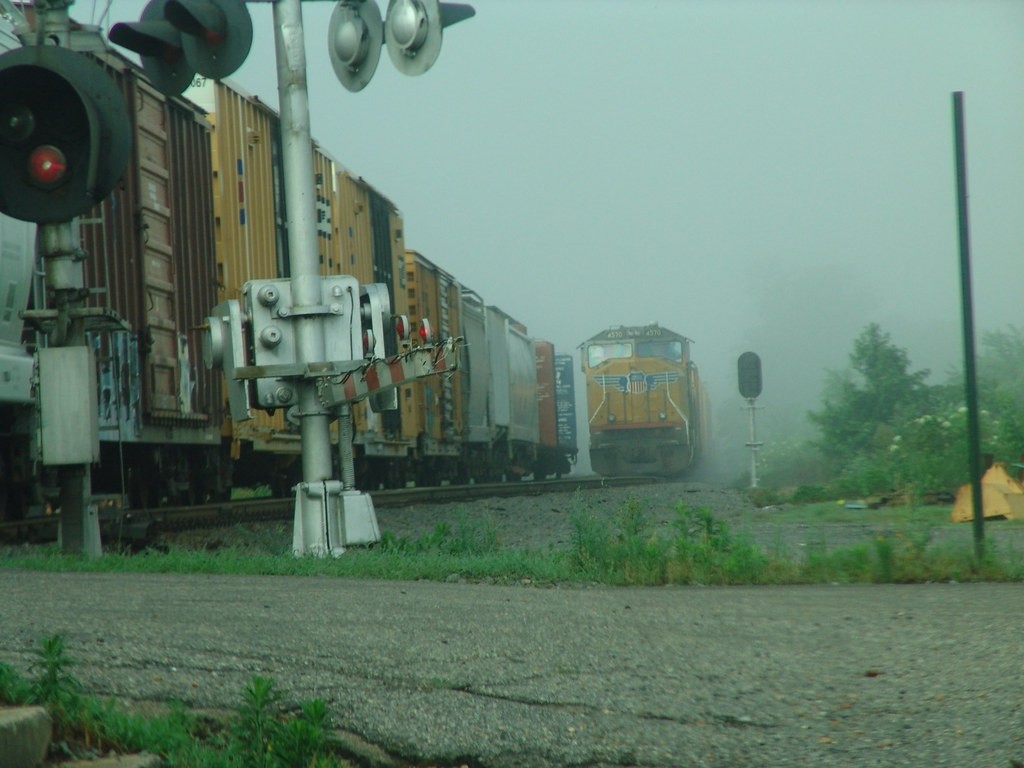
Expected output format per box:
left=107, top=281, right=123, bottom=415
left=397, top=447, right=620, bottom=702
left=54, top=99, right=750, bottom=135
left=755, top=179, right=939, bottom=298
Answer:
left=109, top=1, right=255, bottom=96
left=0, top=45, right=132, bottom=225
left=328, top=1, right=474, bottom=94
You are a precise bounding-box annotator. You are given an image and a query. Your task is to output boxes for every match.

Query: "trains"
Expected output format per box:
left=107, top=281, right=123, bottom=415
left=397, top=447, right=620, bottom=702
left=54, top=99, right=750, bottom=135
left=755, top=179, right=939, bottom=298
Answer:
left=0, top=1, right=579, bottom=524
left=577, top=321, right=715, bottom=480
